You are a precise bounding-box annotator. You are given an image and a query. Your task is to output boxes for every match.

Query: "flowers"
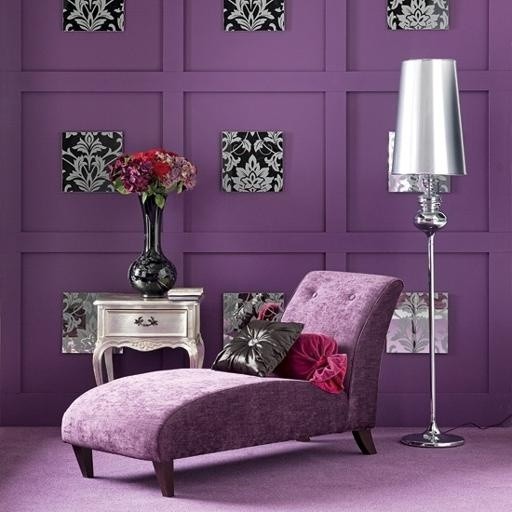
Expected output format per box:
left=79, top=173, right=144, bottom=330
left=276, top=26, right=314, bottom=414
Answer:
left=107, top=147, right=197, bottom=210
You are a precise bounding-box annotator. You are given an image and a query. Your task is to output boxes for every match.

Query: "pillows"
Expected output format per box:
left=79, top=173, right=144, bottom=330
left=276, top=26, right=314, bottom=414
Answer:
left=273, top=332, right=348, bottom=394
left=211, top=319, right=305, bottom=378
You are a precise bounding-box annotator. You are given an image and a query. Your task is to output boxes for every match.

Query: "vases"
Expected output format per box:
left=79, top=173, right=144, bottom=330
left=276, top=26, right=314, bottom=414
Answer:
left=126, top=190, right=177, bottom=299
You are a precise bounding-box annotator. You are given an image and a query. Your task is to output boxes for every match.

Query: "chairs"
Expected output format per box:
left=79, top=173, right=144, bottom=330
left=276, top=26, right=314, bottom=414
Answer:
left=61, top=270, right=406, bottom=498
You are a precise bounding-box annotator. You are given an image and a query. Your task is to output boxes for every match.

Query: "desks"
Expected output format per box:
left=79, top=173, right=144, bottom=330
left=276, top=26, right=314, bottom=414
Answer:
left=91, top=294, right=206, bottom=387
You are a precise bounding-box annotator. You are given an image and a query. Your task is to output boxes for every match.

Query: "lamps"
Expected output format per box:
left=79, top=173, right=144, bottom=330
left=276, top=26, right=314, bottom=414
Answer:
left=391, top=59, right=469, bottom=449
